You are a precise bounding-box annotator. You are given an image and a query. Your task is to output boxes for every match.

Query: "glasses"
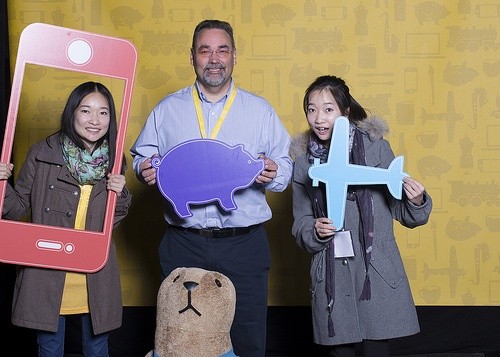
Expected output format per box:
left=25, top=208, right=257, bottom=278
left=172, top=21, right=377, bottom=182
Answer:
left=193, top=48, right=232, bottom=58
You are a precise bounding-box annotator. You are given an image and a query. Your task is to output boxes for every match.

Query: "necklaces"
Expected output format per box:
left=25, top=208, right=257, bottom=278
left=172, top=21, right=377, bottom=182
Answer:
left=193, top=82, right=237, bottom=139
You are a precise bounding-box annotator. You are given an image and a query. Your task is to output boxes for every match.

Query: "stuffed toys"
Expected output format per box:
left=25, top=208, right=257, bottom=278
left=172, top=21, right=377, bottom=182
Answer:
left=144, top=266, right=237, bottom=356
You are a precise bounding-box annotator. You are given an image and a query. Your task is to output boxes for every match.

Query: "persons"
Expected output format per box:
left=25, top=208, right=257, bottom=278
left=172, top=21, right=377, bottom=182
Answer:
left=0, top=80, right=132, bottom=356
left=291, top=74, right=433, bottom=357
left=128, top=20, right=294, bottom=357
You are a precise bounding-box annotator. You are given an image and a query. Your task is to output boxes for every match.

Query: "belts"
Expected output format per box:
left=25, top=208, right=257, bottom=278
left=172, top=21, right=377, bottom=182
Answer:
left=170, top=224, right=262, bottom=238
